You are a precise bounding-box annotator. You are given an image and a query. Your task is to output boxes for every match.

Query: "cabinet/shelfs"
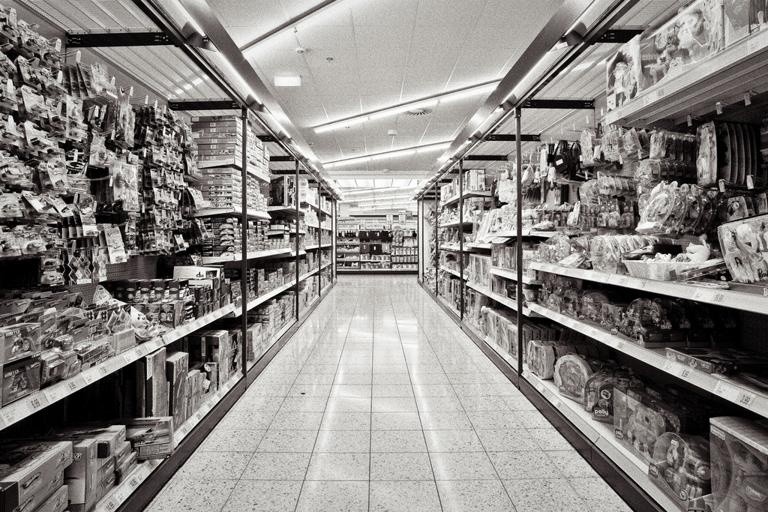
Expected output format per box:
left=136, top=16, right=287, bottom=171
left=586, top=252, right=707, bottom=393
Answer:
left=337, top=215, right=420, bottom=273
left=296, top=148, right=336, bottom=325
left=244, top=100, right=298, bottom=375
left=417, top=107, right=517, bottom=388
left=518, top=0, right=768, bottom=512
left=1, top=0, right=246, bottom=512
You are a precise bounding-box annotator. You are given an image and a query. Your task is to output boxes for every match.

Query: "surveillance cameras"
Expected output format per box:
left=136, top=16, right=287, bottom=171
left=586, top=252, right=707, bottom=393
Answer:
left=295, top=48, right=305, bottom=55
left=309, top=142, right=313, bottom=145
